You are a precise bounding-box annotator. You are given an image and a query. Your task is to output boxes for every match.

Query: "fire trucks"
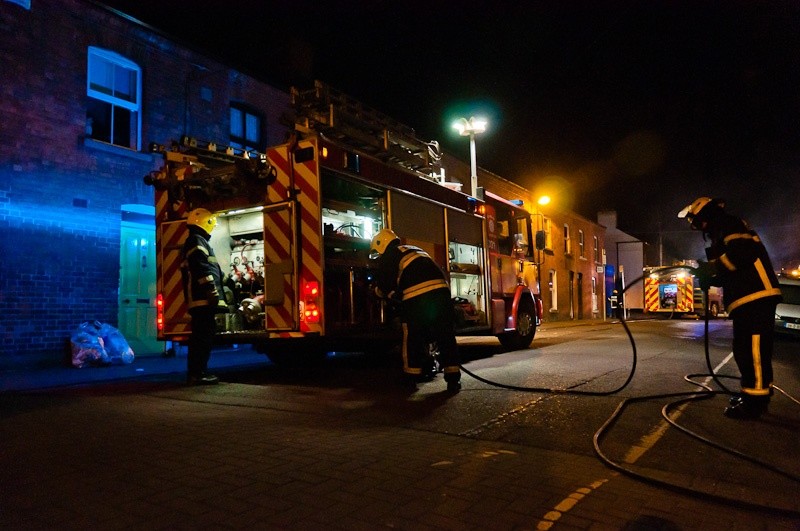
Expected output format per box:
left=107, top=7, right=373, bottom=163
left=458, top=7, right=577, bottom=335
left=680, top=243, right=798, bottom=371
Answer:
left=142, top=79, right=546, bottom=364
left=642, top=259, right=724, bottom=319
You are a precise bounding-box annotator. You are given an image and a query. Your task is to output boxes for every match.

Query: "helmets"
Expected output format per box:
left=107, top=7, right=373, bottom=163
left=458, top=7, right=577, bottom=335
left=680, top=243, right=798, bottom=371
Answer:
left=369, top=230, right=401, bottom=255
left=187, top=208, right=218, bottom=238
left=677, top=197, right=724, bottom=233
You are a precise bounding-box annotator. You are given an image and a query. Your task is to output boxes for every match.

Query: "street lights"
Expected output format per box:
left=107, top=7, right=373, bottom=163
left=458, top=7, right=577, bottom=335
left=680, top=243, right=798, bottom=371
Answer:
left=452, top=115, right=487, bottom=198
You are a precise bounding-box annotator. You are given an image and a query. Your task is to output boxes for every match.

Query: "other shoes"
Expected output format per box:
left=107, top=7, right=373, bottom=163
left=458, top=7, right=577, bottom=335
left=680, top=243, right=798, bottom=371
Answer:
left=447, top=382, right=461, bottom=392
left=724, top=399, right=762, bottom=420
left=194, top=376, right=218, bottom=385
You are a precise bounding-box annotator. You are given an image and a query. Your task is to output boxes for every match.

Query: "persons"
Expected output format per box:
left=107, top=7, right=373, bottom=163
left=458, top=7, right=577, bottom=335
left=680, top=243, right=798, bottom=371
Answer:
left=370, top=228, right=462, bottom=395
left=180, top=208, right=225, bottom=385
left=687, top=197, right=783, bottom=420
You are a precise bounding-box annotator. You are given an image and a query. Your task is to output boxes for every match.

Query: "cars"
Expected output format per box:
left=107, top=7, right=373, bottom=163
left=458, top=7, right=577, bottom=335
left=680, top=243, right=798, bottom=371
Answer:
left=774, top=276, right=800, bottom=335
